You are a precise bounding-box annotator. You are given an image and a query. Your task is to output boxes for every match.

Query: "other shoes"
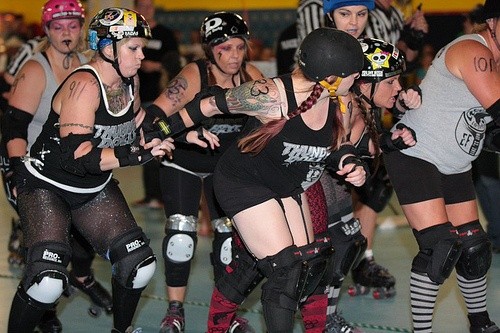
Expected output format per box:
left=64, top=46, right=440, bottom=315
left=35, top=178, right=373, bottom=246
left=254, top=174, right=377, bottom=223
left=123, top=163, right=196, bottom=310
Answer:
left=326, top=316, right=362, bottom=333
left=226, top=318, right=249, bottom=333
left=157, top=307, right=184, bottom=332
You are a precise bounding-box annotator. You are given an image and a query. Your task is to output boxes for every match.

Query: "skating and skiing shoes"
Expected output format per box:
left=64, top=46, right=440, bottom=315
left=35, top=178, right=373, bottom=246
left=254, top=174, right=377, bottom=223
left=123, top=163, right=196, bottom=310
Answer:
left=36, top=314, right=63, bottom=333
left=348, top=258, right=397, bottom=299
left=63, top=270, right=113, bottom=316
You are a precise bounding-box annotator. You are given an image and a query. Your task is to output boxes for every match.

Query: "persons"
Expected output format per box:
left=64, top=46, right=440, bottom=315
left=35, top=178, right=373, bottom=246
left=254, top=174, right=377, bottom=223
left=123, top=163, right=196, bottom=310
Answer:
left=463, top=0, right=500, bottom=255
left=384, top=0, right=500, bottom=333
left=278, top=0, right=432, bottom=333
left=8, top=6, right=175, bottom=333
left=145, top=10, right=264, bottom=333
left=139, top=27, right=366, bottom=333
left=133, top=0, right=213, bottom=237
left=0, top=0, right=112, bottom=319
left=169, top=29, right=275, bottom=68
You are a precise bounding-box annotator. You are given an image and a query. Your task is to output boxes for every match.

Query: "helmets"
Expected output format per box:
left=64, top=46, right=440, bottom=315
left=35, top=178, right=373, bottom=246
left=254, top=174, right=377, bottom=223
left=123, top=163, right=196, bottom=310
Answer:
left=322, top=0, right=375, bottom=14
left=200, top=13, right=249, bottom=49
left=355, top=38, right=406, bottom=82
left=297, top=27, right=364, bottom=81
left=88, top=7, right=152, bottom=50
left=41, top=0, right=85, bottom=27
